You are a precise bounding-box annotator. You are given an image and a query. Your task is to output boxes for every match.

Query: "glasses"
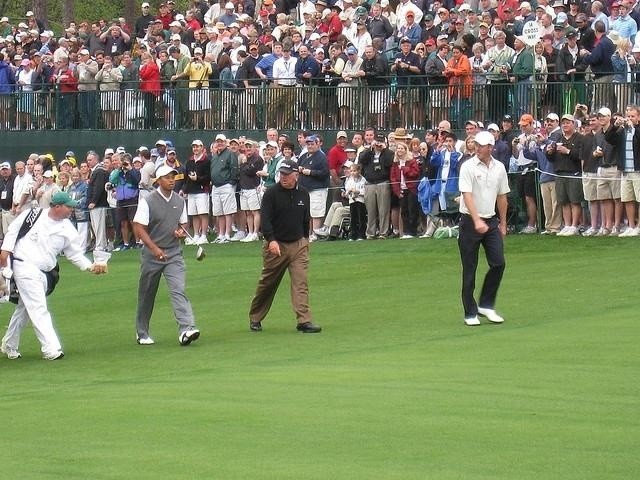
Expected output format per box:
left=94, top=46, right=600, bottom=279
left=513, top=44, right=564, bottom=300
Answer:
left=418, top=47, right=425, bottom=51
left=419, top=148, right=426, bottom=151
left=55, top=61, right=65, bottom=64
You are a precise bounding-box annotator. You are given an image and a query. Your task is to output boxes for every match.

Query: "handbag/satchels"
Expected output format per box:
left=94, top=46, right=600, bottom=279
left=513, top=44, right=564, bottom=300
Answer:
left=8, top=207, right=60, bottom=304
left=231, top=88, right=238, bottom=113
left=417, top=176, right=434, bottom=215
left=104, top=181, right=116, bottom=207
left=189, top=79, right=203, bottom=89
left=536, top=56, right=545, bottom=81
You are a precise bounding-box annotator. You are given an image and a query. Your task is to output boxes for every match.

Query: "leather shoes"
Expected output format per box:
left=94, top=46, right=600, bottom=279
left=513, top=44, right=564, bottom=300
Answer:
left=296, top=323, right=326, bottom=332
left=248, top=318, right=263, bottom=332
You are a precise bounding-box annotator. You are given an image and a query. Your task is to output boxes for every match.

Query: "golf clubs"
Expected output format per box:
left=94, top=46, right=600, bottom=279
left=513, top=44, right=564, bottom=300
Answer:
left=177, top=223, right=206, bottom=262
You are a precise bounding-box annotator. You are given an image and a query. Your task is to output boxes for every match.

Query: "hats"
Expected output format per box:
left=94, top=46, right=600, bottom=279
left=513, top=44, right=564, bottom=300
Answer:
left=50, top=192, right=79, bottom=206
left=479, top=23, right=490, bottom=28
left=79, top=21, right=90, bottom=27
left=346, top=47, right=358, bottom=54
left=215, top=22, right=226, bottom=30
left=339, top=13, right=349, bottom=21
left=315, top=47, right=326, bottom=54
left=33, top=52, right=43, bottom=59
left=156, top=166, right=179, bottom=176
left=439, top=35, right=448, bottom=42
left=279, top=134, right=290, bottom=139
left=66, top=152, right=75, bottom=157
left=356, top=21, right=365, bottom=25
left=166, top=0, right=178, bottom=6
left=438, top=6, right=450, bottom=16
left=619, top=3, right=629, bottom=10
left=227, top=22, right=240, bottom=29
left=314, top=0, right=328, bottom=7
left=519, top=114, right=533, bottom=125
left=46, top=154, right=55, bottom=161
left=450, top=19, right=456, bottom=23
left=282, top=46, right=291, bottom=51
left=455, top=19, right=464, bottom=24
left=259, top=11, right=268, bottom=16
left=250, top=45, right=259, bottom=51
left=474, top=122, right=485, bottom=128
left=392, top=128, right=412, bottom=139
left=556, top=11, right=567, bottom=22
left=245, top=140, right=257, bottom=145
left=309, top=32, right=319, bottom=41
left=173, top=35, right=184, bottom=41
left=514, top=34, right=527, bottom=44
left=155, top=140, right=166, bottom=147
left=29, top=153, right=40, bottom=159
left=64, top=27, right=76, bottom=34
left=61, top=160, right=70, bottom=166
left=150, top=148, right=160, bottom=157
left=40, top=32, right=50, bottom=37
left=342, top=160, right=356, bottom=169
left=138, top=43, right=147, bottom=49
left=321, top=7, right=332, bottom=19
left=424, top=15, right=434, bottom=21
left=141, top=2, right=151, bottom=9
left=25, top=11, right=34, bottom=17
left=165, top=141, right=176, bottom=147
left=216, top=135, right=227, bottom=141
left=5, top=35, right=14, bottom=41
left=117, top=146, right=127, bottom=153
left=104, top=148, right=116, bottom=155
left=30, top=30, right=39, bottom=34
left=425, top=38, right=435, bottom=46
left=322, top=59, right=334, bottom=67
left=222, top=38, right=230, bottom=44
left=502, top=6, right=513, bottom=13
left=206, top=27, right=220, bottom=34
left=535, top=5, right=547, bottom=11
left=224, top=3, right=236, bottom=10
left=518, top=2, right=532, bottom=12
left=608, top=3, right=621, bottom=11
left=459, top=5, right=473, bottom=12
left=449, top=7, right=457, bottom=13
left=575, top=13, right=588, bottom=23
left=277, top=161, right=300, bottom=175
left=68, top=156, right=76, bottom=165
left=168, top=150, right=177, bottom=154
left=42, top=171, right=54, bottom=177
left=0, top=162, right=12, bottom=171
left=18, top=22, right=31, bottom=29
left=194, top=46, right=204, bottom=55
left=159, top=3, right=168, bottom=8
left=552, top=1, right=566, bottom=8
left=59, top=38, right=70, bottom=45
left=540, top=34, right=555, bottom=40
left=466, top=120, right=476, bottom=128
left=79, top=49, right=91, bottom=55
left=176, top=14, right=185, bottom=21
left=229, top=139, right=239, bottom=144
left=14, top=55, right=23, bottom=60
left=45, top=30, right=54, bottom=36
left=570, top=0, right=578, bottom=5
left=502, top=115, right=513, bottom=123
left=487, top=123, right=500, bottom=130
left=21, top=59, right=31, bottom=65
left=190, top=139, right=204, bottom=146
left=345, top=146, right=357, bottom=153
left=302, top=8, right=314, bottom=15
left=264, top=0, right=276, bottom=5
left=132, top=157, right=144, bottom=164
left=168, top=21, right=185, bottom=30
left=266, top=142, right=278, bottom=148
left=399, top=37, right=412, bottom=43
left=606, top=30, right=623, bottom=44
left=136, top=146, right=148, bottom=151
left=357, top=7, right=368, bottom=17
left=562, top=114, right=576, bottom=122
left=18, top=32, right=28, bottom=37
left=155, top=20, right=164, bottom=24
left=306, top=137, right=318, bottom=143
left=0, top=17, right=9, bottom=23
left=405, top=11, right=416, bottom=17
left=546, top=113, right=560, bottom=121
left=168, top=47, right=180, bottom=57
left=597, top=108, right=612, bottom=117
left=632, top=45, right=639, bottom=53
left=378, top=1, right=390, bottom=7
left=374, top=133, right=385, bottom=142
left=566, top=31, right=578, bottom=39
left=337, top=131, right=348, bottom=138
left=230, top=36, right=244, bottom=44
left=475, top=133, right=498, bottom=148
left=305, top=24, right=313, bottom=31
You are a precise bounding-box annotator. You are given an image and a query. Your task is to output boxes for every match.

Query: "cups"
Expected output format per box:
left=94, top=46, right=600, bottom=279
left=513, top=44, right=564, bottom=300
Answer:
left=555, top=143, right=563, bottom=150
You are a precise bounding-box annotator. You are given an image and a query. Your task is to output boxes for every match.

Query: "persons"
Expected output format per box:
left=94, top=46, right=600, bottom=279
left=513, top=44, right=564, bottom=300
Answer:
left=132, top=166, right=201, bottom=347
left=0, top=102, right=639, bottom=255
left=0, top=190, right=108, bottom=361
left=1, top=1, right=640, bottom=128
left=457, top=130, right=511, bottom=327
left=248, top=158, right=322, bottom=334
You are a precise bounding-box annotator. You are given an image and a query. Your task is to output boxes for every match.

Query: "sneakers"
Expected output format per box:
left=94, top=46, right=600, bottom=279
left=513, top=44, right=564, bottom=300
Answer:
left=218, top=237, right=231, bottom=244
left=378, top=233, right=390, bottom=240
left=389, top=232, right=400, bottom=240
left=211, top=237, right=225, bottom=244
left=231, top=231, right=247, bottom=242
left=563, top=226, right=579, bottom=236
left=594, top=226, right=610, bottom=238
left=418, top=232, right=433, bottom=239
left=115, top=240, right=122, bottom=252
left=135, top=332, right=155, bottom=345
left=626, top=227, right=639, bottom=237
left=347, top=237, right=354, bottom=242
left=133, top=241, right=140, bottom=251
left=121, top=240, right=129, bottom=252
left=609, top=227, right=621, bottom=237
left=556, top=225, right=571, bottom=237
left=541, top=226, right=549, bottom=235
left=367, top=234, right=377, bottom=241
left=179, top=327, right=201, bottom=346
left=106, top=240, right=114, bottom=251
left=548, top=229, right=560, bottom=235
left=519, top=224, right=538, bottom=234
left=38, top=346, right=68, bottom=362
left=187, top=233, right=200, bottom=246
left=356, top=237, right=365, bottom=242
left=463, top=313, right=481, bottom=325
left=617, top=227, right=634, bottom=238
left=478, top=307, right=504, bottom=325
left=313, top=226, right=331, bottom=238
left=400, top=234, right=414, bottom=239
left=240, top=232, right=260, bottom=242
left=309, top=234, right=318, bottom=242
left=1, top=344, right=20, bottom=359
left=193, top=234, right=210, bottom=245
left=582, top=227, right=596, bottom=237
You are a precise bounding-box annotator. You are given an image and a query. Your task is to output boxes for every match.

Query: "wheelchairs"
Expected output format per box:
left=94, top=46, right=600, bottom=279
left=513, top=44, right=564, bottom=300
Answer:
left=315, top=213, right=352, bottom=243
